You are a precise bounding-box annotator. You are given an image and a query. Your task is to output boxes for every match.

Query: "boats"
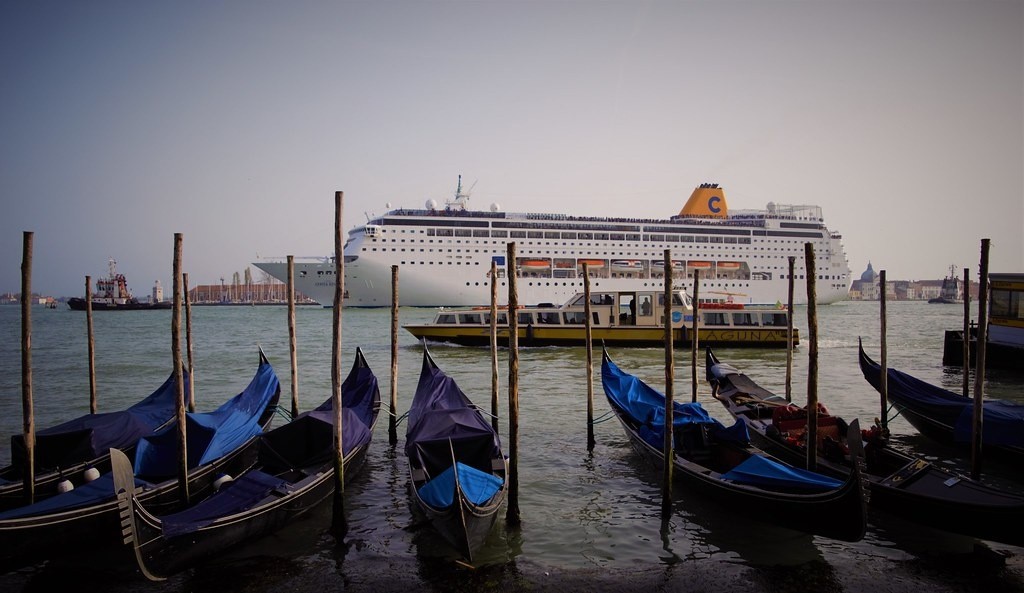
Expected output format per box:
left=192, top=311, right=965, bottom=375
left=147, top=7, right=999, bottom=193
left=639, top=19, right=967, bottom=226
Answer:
left=611, top=261, right=644, bottom=273
left=520, top=260, right=551, bottom=272
left=67, top=258, right=173, bottom=311
left=404, top=337, right=509, bottom=562
left=601, top=337, right=873, bottom=542
left=706, top=349, right=1024, bottom=548
left=45, top=300, right=58, bottom=309
left=944, top=273, right=1024, bottom=364
left=858, top=335, right=1024, bottom=464
left=110, top=347, right=381, bottom=581
left=578, top=260, right=606, bottom=269
left=927, top=265, right=973, bottom=304
left=716, top=262, right=740, bottom=271
left=0, top=360, right=190, bottom=499
left=0, top=348, right=281, bottom=555
left=652, top=262, right=684, bottom=274
left=687, top=262, right=711, bottom=272
left=251, top=174, right=854, bottom=309
left=401, top=289, right=801, bottom=367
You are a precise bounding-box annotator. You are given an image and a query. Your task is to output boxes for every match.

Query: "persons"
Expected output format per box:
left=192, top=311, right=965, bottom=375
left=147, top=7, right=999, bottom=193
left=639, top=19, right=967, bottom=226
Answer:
left=630, top=297, right=636, bottom=316
left=641, top=297, right=650, bottom=316
